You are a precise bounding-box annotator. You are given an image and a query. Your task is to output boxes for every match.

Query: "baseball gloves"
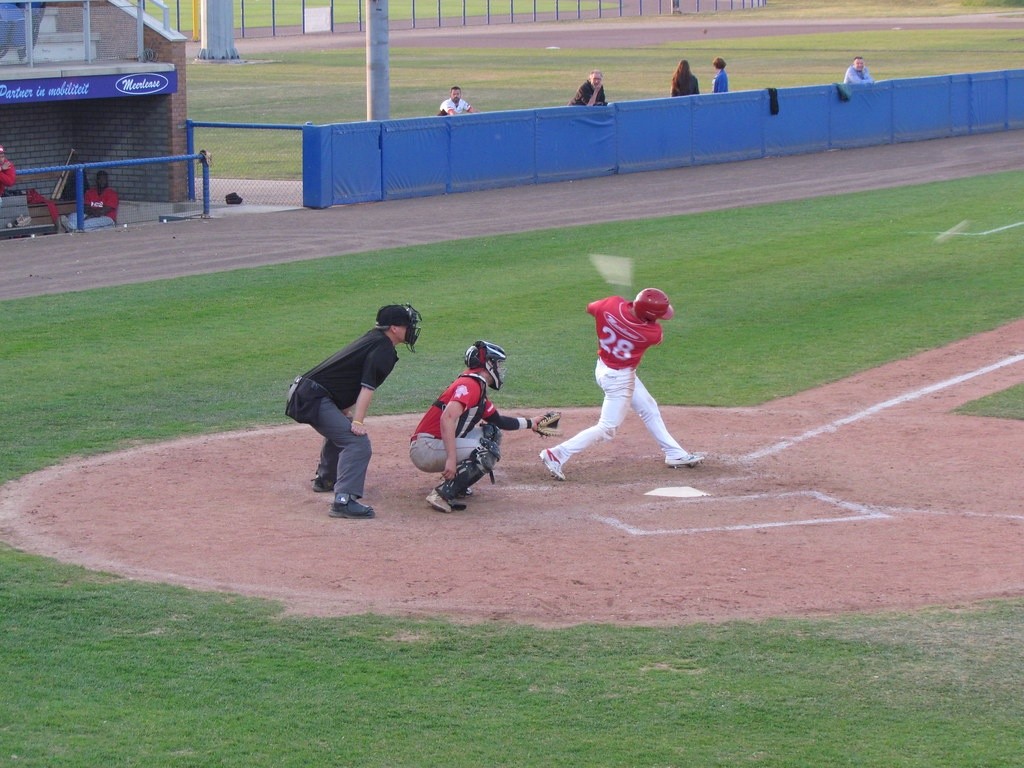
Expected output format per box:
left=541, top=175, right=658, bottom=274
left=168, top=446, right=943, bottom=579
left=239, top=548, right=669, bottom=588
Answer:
left=537, top=410, right=563, bottom=440
left=226, top=192, right=243, bottom=204
left=15, top=214, right=32, bottom=227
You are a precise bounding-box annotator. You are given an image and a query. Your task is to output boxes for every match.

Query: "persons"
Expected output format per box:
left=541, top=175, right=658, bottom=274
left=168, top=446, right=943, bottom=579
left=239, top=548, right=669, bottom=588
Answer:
left=68, top=171, right=119, bottom=229
left=440, top=86, right=480, bottom=116
left=410, top=340, right=545, bottom=514
left=539, top=287, right=706, bottom=481
left=567, top=70, right=608, bottom=107
left=286, top=302, right=423, bottom=519
left=670, top=60, right=700, bottom=97
left=844, top=56, right=875, bottom=85
left=0, top=145, right=16, bottom=197
left=712, top=56, right=728, bottom=94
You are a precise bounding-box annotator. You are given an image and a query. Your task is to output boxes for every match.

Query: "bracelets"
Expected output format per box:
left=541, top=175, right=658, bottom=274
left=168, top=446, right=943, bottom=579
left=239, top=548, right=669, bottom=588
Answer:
left=353, top=420, right=364, bottom=426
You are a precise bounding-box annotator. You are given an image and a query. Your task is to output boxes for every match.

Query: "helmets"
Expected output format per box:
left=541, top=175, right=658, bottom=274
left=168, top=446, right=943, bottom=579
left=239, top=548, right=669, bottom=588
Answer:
left=465, top=341, right=507, bottom=390
left=634, top=288, right=673, bottom=320
left=376, top=303, right=422, bottom=346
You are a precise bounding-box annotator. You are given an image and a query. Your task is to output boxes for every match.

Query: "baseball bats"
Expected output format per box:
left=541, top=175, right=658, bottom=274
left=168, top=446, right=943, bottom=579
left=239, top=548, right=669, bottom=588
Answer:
left=50, top=148, right=76, bottom=200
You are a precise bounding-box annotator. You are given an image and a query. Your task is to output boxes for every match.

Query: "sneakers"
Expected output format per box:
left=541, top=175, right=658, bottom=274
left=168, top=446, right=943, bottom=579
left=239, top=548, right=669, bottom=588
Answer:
left=665, top=454, right=705, bottom=469
left=328, top=499, right=375, bottom=518
left=539, top=449, right=566, bottom=481
left=426, top=488, right=451, bottom=513
left=311, top=476, right=336, bottom=491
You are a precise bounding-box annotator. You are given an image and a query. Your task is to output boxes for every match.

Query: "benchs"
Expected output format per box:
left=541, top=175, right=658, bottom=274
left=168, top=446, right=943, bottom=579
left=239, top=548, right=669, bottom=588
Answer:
left=0, top=31, right=100, bottom=66
left=0, top=194, right=59, bottom=240
